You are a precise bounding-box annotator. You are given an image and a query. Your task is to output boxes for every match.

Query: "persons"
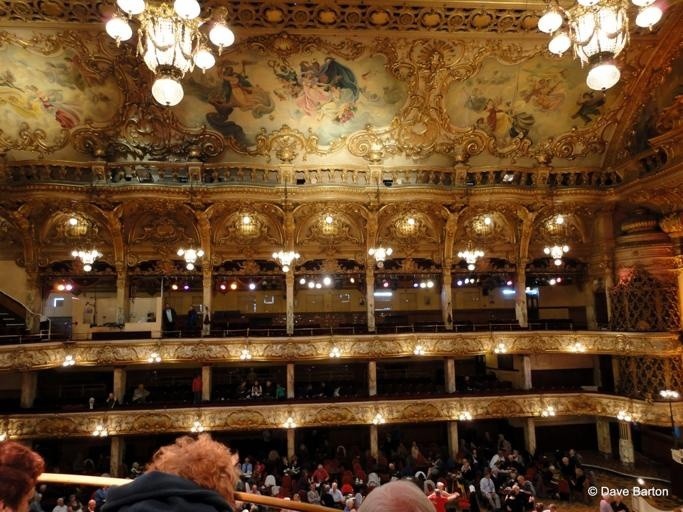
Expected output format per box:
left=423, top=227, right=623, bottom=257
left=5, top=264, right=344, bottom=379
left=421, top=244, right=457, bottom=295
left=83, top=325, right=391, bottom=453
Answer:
left=639, top=376, right=660, bottom=402
left=634, top=313, right=649, bottom=332
left=0, top=301, right=630, bottom=512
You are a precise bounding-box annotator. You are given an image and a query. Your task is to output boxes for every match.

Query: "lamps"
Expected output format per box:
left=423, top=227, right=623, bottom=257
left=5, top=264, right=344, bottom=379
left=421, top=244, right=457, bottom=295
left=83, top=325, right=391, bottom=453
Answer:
left=105, top=0, right=236, bottom=106
left=71, top=173, right=570, bottom=272
left=537, top=0, right=663, bottom=91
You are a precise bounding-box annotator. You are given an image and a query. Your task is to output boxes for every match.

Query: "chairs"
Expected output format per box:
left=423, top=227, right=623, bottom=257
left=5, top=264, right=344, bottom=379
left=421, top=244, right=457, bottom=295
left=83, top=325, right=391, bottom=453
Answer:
left=299, top=458, right=571, bottom=511
